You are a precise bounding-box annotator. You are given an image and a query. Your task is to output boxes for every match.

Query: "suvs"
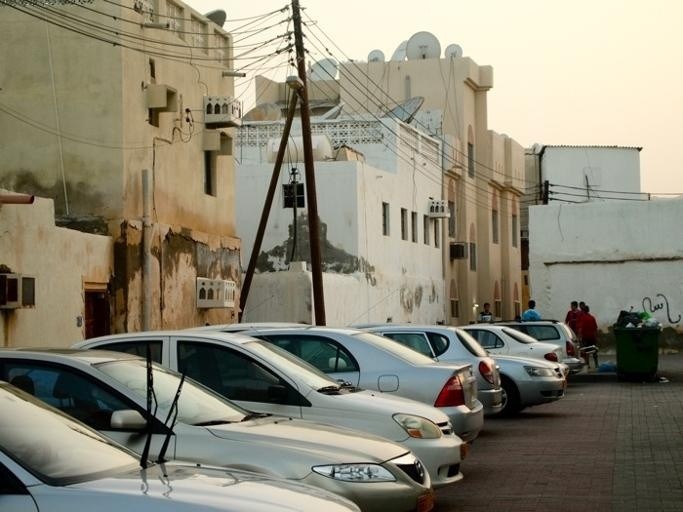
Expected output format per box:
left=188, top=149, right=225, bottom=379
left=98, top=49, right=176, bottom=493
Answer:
left=475, top=320, right=585, bottom=376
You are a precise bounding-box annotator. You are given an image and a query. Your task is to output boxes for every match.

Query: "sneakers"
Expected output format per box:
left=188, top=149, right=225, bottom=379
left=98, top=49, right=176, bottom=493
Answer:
left=595, top=362, right=600, bottom=369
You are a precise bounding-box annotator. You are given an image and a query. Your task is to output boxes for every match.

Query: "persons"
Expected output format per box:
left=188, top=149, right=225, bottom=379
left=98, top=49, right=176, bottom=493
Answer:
left=575, top=306, right=601, bottom=369
left=521, top=300, right=541, bottom=320
left=564, top=301, right=581, bottom=334
left=476, top=303, right=492, bottom=322
left=575, top=302, right=587, bottom=316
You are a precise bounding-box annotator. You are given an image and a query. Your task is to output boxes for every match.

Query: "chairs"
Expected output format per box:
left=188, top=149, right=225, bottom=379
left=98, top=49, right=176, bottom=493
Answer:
left=52, top=372, right=88, bottom=421
left=10, top=376, right=36, bottom=396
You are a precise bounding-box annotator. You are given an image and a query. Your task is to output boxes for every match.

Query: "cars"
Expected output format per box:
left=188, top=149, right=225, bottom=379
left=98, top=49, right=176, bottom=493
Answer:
left=360, top=327, right=509, bottom=417
left=0, top=382, right=363, bottom=512
left=169, top=325, right=484, bottom=452
left=387, top=332, right=567, bottom=417
left=15, top=332, right=466, bottom=490
left=0, top=348, right=434, bottom=512
left=456, top=322, right=569, bottom=381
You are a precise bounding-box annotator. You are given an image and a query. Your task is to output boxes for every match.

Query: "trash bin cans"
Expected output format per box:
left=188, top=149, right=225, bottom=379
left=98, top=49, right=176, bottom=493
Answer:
left=615, top=322, right=658, bottom=380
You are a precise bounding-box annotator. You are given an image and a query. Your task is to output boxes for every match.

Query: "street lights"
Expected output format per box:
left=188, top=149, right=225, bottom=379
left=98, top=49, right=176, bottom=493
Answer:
left=286, top=75, right=326, bottom=326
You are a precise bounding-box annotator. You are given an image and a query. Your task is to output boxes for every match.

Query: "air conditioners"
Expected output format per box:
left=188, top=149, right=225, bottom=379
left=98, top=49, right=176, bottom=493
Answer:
left=0, top=273, right=37, bottom=310
